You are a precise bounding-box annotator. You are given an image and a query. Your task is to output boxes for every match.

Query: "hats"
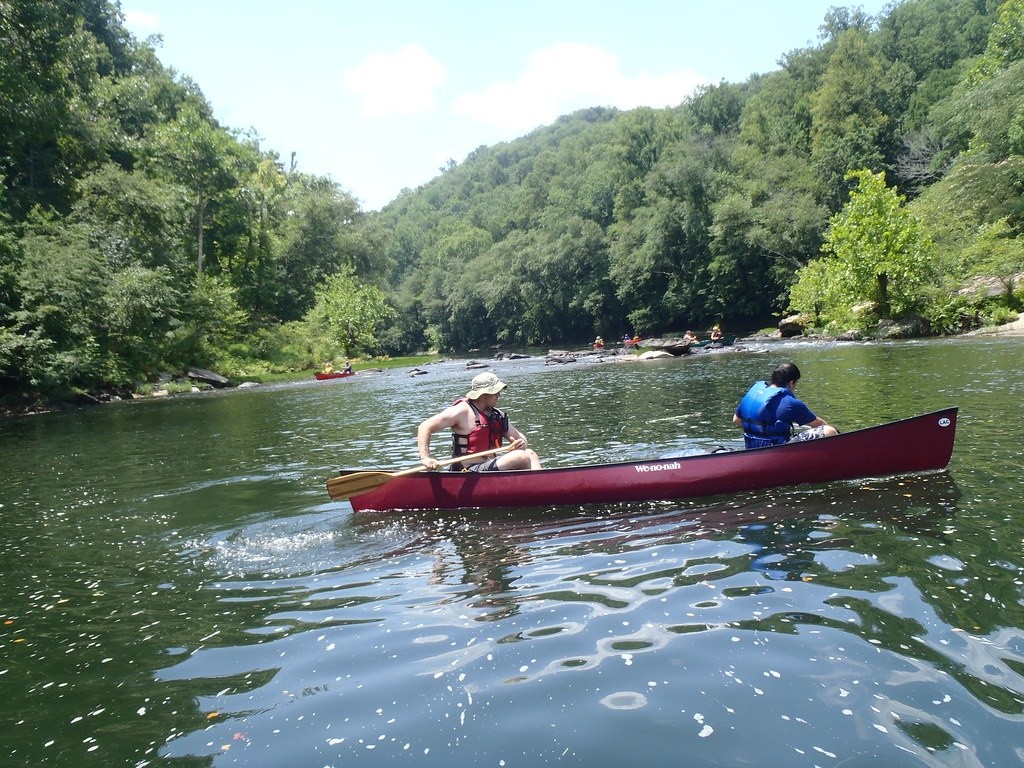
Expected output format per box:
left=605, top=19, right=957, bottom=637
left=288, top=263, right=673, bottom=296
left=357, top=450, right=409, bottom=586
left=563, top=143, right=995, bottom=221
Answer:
left=466, top=372, right=508, bottom=400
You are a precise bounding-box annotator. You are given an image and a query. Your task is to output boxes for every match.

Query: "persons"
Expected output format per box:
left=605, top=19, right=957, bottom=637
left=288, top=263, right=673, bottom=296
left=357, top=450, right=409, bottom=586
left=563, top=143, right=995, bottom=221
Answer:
left=732, top=362, right=838, bottom=449
left=418, top=373, right=541, bottom=469
left=341, top=361, right=351, bottom=373
left=711, top=326, right=724, bottom=340
left=624, top=332, right=639, bottom=339
left=683, top=328, right=696, bottom=341
left=322, top=363, right=333, bottom=374
left=594, top=336, right=603, bottom=344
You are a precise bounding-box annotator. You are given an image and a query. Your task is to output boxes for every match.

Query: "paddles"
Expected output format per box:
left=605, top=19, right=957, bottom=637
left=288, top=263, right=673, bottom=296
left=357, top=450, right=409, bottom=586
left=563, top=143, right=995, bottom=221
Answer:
left=325, top=439, right=523, bottom=503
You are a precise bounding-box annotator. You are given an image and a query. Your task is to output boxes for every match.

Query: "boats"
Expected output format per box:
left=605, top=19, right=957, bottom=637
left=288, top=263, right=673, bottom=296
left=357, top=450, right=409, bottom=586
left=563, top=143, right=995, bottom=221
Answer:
left=338, top=406, right=958, bottom=514
left=618, top=338, right=641, bottom=345
left=592, top=342, right=603, bottom=349
left=314, top=372, right=353, bottom=380
left=689, top=337, right=729, bottom=347
